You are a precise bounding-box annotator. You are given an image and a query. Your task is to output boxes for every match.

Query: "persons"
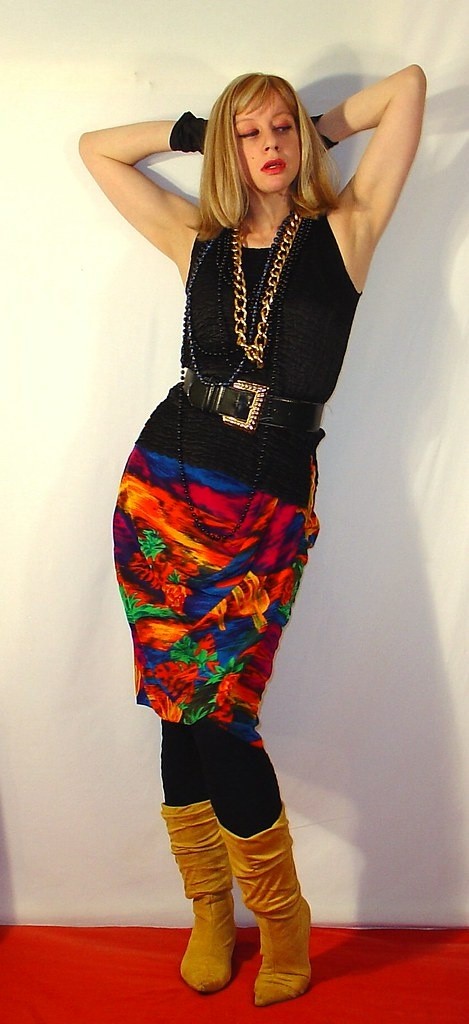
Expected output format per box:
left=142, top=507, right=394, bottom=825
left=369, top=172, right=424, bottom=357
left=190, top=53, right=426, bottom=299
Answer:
left=79, top=64, right=429, bottom=1006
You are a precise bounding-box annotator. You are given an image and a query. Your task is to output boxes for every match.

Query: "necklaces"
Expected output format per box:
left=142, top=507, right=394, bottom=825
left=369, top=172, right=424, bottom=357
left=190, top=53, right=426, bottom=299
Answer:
left=187, top=211, right=300, bottom=387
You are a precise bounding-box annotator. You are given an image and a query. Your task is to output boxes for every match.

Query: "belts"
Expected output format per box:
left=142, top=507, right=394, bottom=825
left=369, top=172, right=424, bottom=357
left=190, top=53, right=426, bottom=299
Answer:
left=183, top=368, right=324, bottom=434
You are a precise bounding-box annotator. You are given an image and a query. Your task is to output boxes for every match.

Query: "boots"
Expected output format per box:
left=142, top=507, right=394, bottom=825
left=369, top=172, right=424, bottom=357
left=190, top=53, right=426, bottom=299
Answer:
left=160, top=798, right=236, bottom=992
left=217, top=800, right=310, bottom=1007
